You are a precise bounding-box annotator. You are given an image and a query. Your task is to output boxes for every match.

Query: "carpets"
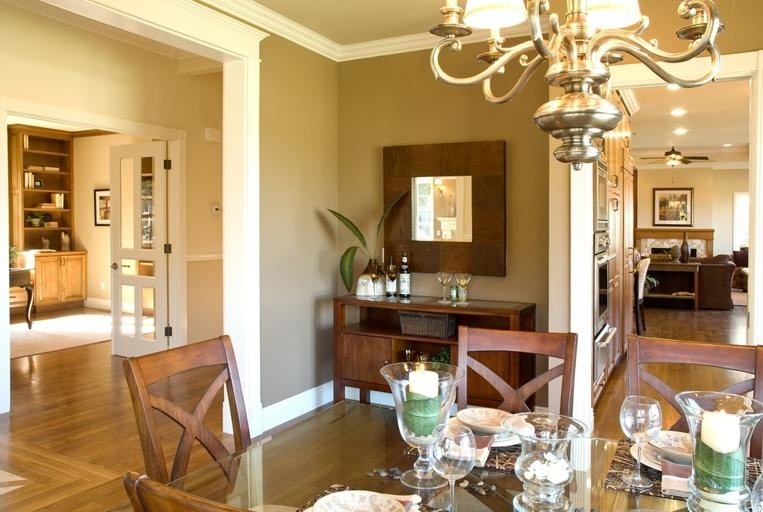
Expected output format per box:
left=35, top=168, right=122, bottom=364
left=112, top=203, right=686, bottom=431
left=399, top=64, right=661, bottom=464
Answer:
left=731, top=288, right=748, bottom=306
left=10, top=307, right=154, bottom=360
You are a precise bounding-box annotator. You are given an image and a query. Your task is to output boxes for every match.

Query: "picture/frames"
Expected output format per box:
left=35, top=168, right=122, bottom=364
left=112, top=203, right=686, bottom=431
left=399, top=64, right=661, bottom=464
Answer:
left=94, top=188, right=111, bottom=226
left=652, top=187, right=694, bottom=227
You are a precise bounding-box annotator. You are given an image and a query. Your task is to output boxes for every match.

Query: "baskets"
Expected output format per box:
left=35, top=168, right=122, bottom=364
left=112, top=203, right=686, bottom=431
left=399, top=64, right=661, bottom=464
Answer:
left=398, top=310, right=456, bottom=340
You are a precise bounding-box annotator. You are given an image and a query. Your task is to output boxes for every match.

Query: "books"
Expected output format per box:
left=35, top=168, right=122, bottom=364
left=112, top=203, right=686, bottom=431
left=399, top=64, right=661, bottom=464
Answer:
left=36, top=192, right=64, bottom=210
left=23, top=134, right=29, bottom=149
left=44, top=166, right=61, bottom=172
left=24, top=166, right=42, bottom=189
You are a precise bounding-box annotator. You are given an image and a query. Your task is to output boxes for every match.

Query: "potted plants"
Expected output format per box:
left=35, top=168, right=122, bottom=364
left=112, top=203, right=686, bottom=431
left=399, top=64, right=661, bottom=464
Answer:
left=326, top=189, right=408, bottom=302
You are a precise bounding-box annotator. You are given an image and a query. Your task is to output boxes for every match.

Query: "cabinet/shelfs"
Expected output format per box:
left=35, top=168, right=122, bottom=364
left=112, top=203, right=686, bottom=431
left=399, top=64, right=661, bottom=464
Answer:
left=622, top=102, right=636, bottom=354
left=8, top=124, right=73, bottom=251
left=608, top=89, right=622, bottom=370
left=12, top=253, right=86, bottom=312
left=332, top=293, right=536, bottom=405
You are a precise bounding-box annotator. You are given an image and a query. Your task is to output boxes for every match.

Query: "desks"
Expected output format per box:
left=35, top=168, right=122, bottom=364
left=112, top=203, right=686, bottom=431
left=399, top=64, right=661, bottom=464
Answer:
left=643, top=261, right=701, bottom=308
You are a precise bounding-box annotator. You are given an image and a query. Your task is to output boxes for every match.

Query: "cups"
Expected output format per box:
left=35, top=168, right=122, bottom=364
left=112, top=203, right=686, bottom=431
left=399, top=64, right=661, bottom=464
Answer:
left=750, top=473, right=762, bottom=511
left=674, top=391, right=762, bottom=511
left=504, top=411, right=589, bottom=512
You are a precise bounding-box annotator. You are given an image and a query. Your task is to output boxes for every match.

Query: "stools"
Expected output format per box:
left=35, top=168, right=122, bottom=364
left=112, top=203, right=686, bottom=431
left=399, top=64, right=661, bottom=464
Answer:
left=9, top=268, right=37, bottom=329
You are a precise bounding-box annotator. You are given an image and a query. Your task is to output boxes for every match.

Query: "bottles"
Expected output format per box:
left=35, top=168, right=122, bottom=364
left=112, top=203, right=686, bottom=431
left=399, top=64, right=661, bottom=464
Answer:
left=400, top=251, right=411, bottom=297
left=386, top=254, right=397, bottom=297
left=449, top=273, right=459, bottom=301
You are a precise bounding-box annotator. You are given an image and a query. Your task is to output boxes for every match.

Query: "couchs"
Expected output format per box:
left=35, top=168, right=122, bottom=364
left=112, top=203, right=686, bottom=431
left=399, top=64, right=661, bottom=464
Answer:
left=699, top=254, right=737, bottom=310
left=729, top=247, right=748, bottom=292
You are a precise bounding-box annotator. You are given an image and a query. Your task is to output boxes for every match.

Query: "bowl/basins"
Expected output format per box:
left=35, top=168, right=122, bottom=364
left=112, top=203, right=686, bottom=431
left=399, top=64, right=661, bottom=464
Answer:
left=457, top=406, right=512, bottom=434
left=646, top=430, right=693, bottom=465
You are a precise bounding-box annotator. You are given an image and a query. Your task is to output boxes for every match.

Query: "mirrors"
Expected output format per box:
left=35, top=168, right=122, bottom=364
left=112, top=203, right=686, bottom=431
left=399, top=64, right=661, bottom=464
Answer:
left=383, top=141, right=506, bottom=275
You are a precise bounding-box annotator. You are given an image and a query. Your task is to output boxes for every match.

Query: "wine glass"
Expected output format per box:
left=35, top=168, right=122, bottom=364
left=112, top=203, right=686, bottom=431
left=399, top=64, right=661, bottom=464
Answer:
left=618, top=394, right=663, bottom=488
left=380, top=362, right=465, bottom=491
left=367, top=264, right=382, bottom=300
left=436, top=271, right=452, bottom=304
left=429, top=423, right=477, bottom=512
left=454, top=272, right=472, bottom=303
left=386, top=268, right=400, bottom=300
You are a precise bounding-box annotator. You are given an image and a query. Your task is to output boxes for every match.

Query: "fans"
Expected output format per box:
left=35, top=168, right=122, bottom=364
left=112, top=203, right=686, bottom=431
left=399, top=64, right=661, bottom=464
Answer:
left=640, top=146, right=708, bottom=165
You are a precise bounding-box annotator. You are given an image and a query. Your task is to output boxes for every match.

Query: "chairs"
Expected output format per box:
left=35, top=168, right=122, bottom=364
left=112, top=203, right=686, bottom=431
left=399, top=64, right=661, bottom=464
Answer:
left=122, top=335, right=252, bottom=485
left=632, top=258, right=650, bottom=335
left=627, top=335, right=763, bottom=467
left=456, top=326, right=578, bottom=429
left=124, top=471, right=253, bottom=512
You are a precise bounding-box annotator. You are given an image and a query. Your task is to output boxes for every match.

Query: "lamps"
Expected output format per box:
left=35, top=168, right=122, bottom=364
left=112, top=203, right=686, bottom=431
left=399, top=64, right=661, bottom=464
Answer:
left=666, top=146, right=681, bottom=184
left=426, top=0, right=726, bottom=172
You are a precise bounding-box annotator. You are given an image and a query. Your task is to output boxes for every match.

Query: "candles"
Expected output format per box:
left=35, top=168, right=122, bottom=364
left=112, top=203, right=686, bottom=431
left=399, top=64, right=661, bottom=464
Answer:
left=408, top=365, right=439, bottom=403
left=702, top=408, right=740, bottom=455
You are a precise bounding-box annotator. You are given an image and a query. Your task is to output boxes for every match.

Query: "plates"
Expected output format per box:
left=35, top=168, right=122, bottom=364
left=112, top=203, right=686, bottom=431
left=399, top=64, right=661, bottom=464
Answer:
left=450, top=415, right=521, bottom=448
left=311, top=489, right=406, bottom=512
left=630, top=441, right=662, bottom=472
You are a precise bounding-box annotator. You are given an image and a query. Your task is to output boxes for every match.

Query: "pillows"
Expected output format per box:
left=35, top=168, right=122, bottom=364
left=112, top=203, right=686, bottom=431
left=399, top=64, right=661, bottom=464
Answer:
left=733, top=251, right=748, bottom=267
